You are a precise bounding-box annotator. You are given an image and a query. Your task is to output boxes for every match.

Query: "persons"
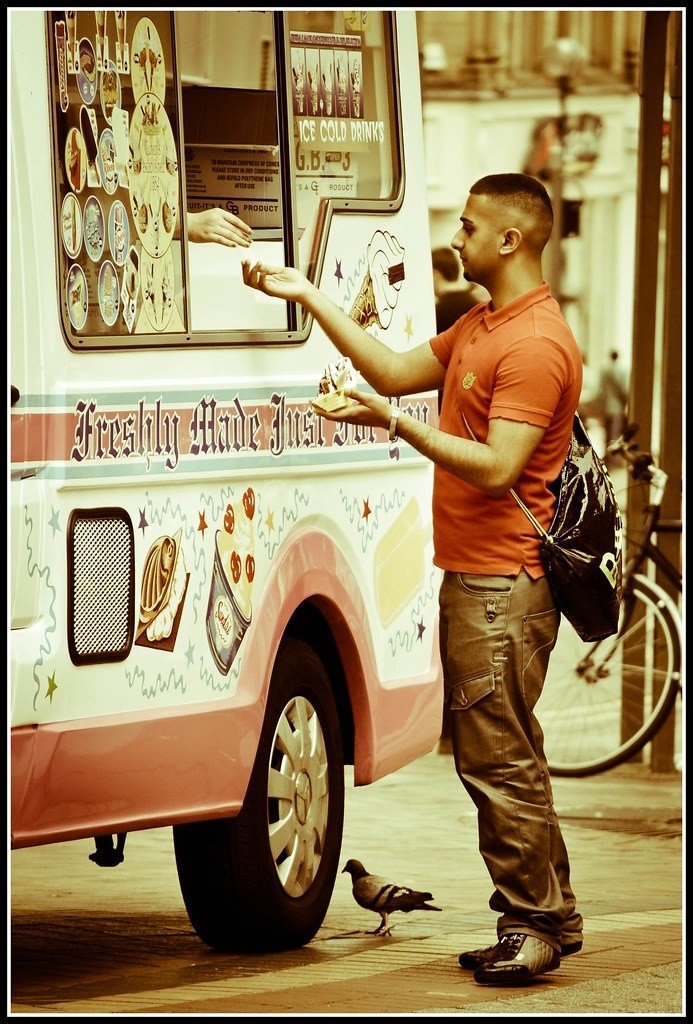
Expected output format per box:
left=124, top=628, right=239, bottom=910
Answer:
left=603, top=351, right=626, bottom=467
left=240, top=172, right=585, bottom=985
left=431, top=246, right=491, bottom=416
left=187, top=207, right=254, bottom=248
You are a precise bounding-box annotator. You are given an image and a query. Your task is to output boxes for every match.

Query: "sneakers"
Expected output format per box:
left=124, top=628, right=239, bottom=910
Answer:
left=474, top=931, right=563, bottom=986
left=459, top=934, right=582, bottom=971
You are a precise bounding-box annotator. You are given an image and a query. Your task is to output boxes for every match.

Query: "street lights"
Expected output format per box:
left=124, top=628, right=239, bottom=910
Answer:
left=541, top=36, right=590, bottom=304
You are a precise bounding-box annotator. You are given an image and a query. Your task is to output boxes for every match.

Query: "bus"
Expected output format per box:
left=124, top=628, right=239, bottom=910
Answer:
left=7, top=7, right=443, bottom=953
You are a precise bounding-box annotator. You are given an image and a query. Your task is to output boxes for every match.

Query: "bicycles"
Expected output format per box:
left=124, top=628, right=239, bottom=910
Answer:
left=547, top=421, right=682, bottom=778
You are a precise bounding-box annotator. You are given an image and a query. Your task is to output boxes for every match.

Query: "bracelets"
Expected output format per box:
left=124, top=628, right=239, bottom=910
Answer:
left=389, top=407, right=399, bottom=442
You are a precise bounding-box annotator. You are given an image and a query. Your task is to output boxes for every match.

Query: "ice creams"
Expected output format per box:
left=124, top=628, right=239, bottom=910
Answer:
left=291, top=48, right=360, bottom=117
left=310, top=357, right=358, bottom=412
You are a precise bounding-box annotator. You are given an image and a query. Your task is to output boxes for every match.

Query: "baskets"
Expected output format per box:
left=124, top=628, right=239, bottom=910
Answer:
left=611, top=482, right=649, bottom=564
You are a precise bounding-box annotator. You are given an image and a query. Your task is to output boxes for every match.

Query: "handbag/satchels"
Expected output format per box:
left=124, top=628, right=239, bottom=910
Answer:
left=535, top=441, right=624, bottom=642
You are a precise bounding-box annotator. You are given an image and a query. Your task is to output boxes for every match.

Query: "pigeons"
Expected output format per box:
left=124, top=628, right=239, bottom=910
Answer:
left=341, top=859, right=442, bottom=939
